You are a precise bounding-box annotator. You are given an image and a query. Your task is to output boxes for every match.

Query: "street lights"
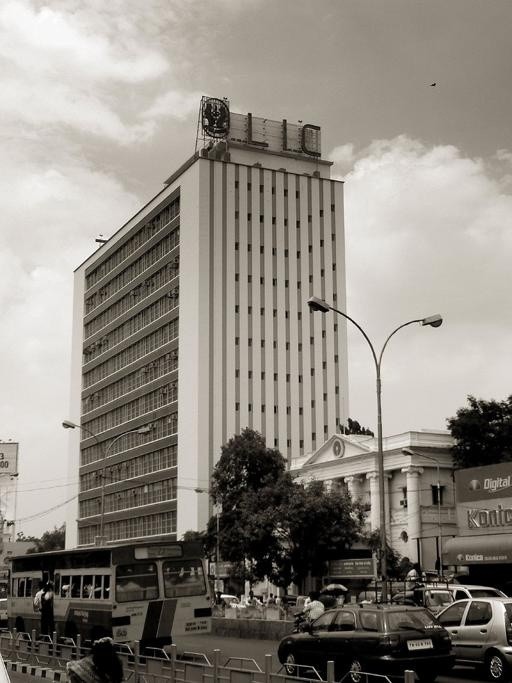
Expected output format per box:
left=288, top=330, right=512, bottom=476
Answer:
left=194, top=487, right=224, bottom=604
left=307, top=296, right=444, bottom=606
left=402, top=446, right=444, bottom=584
left=62, top=419, right=152, bottom=546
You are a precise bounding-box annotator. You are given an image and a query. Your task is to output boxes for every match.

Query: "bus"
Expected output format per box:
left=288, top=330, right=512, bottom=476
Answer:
left=6, top=538, right=216, bottom=660
left=0, top=565, right=10, bottom=628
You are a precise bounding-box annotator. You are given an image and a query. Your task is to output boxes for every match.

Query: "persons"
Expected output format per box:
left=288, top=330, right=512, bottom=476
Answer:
left=215, top=592, right=228, bottom=608
left=245, top=591, right=264, bottom=608
left=67, top=624, right=125, bottom=682
left=34, top=580, right=56, bottom=642
left=334, top=591, right=345, bottom=606
left=268, top=593, right=284, bottom=606
left=294, top=590, right=325, bottom=633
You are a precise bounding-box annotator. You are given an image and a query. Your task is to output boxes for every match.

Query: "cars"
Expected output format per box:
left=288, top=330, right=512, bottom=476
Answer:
left=218, top=584, right=512, bottom=682
